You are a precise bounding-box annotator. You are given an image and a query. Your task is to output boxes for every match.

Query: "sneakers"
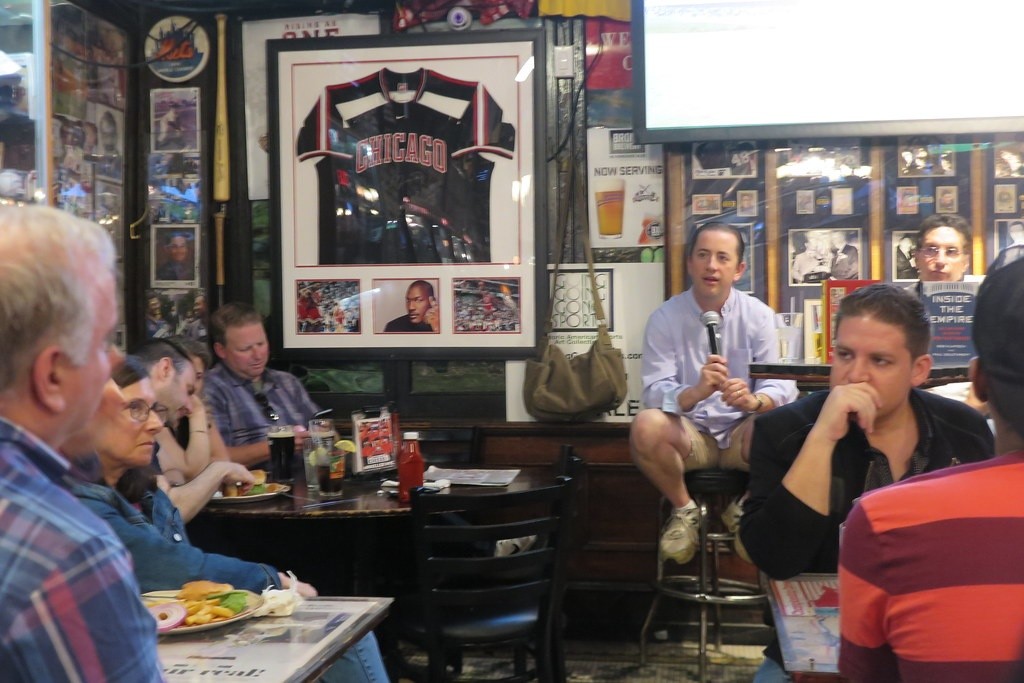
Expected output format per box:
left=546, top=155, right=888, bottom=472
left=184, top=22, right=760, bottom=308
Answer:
left=659, top=505, right=702, bottom=566
left=723, top=496, right=756, bottom=567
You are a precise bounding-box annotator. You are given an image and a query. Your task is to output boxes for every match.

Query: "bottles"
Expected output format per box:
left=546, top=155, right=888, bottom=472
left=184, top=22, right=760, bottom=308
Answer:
left=397, top=431, right=424, bottom=504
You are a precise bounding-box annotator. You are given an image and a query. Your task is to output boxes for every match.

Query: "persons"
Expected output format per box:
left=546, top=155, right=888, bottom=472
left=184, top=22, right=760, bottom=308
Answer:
left=47, top=26, right=211, bottom=342
left=629, top=221, right=799, bottom=565
left=684, top=138, right=1024, bottom=367
left=0, top=204, right=391, bottom=683
left=453, top=280, right=521, bottom=332
left=736, top=282, right=997, bottom=683
left=382, top=279, right=441, bottom=332
left=298, top=281, right=359, bottom=333
left=835, top=257, right=1024, bottom=683
left=899, top=213, right=983, bottom=387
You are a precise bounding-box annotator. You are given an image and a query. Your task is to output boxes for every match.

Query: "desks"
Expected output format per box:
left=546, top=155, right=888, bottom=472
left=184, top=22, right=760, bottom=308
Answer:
left=195, top=462, right=566, bottom=600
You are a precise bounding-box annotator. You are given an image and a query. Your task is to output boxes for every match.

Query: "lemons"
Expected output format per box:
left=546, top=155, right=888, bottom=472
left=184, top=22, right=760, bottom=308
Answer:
left=334, top=440, right=356, bottom=453
left=309, top=447, right=328, bottom=466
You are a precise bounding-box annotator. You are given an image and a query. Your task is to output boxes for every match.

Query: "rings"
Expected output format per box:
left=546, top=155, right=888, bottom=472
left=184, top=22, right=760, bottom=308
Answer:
left=737, top=391, right=742, bottom=396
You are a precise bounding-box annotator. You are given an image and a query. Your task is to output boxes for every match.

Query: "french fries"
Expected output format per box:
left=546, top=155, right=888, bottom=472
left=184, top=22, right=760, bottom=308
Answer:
left=182, top=598, right=234, bottom=624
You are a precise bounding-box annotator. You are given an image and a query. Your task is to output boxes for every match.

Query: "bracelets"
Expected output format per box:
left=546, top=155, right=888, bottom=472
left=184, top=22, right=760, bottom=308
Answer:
left=161, top=467, right=182, bottom=474
left=752, top=393, right=765, bottom=412
left=189, top=429, right=209, bottom=434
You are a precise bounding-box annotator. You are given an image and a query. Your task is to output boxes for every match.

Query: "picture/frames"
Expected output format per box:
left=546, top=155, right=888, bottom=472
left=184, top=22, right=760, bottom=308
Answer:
left=266, top=29, right=550, bottom=361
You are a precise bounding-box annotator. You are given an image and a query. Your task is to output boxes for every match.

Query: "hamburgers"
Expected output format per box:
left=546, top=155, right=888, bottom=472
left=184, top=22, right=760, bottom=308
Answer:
left=236, top=469, right=267, bottom=496
left=176, top=581, right=249, bottom=615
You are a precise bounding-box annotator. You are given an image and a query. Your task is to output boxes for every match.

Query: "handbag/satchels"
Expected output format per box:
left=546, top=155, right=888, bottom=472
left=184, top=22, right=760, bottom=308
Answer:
left=522, top=324, right=628, bottom=423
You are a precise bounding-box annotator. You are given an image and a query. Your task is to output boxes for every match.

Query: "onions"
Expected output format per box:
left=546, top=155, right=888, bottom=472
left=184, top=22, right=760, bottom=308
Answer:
left=146, top=603, right=187, bottom=631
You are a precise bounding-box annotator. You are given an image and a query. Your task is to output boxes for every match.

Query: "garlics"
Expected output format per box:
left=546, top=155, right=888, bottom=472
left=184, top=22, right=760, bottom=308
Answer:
left=254, top=588, right=298, bottom=617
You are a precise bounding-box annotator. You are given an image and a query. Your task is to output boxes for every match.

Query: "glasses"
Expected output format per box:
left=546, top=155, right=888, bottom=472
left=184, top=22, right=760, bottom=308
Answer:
left=124, top=399, right=169, bottom=424
left=917, top=246, right=967, bottom=257
left=985, top=244, right=1024, bottom=284
left=255, top=391, right=279, bottom=421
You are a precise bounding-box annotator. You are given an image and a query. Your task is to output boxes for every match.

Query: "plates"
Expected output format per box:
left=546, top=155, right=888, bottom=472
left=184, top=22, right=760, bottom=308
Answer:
left=213, top=483, right=291, bottom=504
left=141, top=589, right=265, bottom=636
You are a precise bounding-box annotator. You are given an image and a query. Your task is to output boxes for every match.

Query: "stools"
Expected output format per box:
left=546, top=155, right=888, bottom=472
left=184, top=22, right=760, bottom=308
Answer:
left=639, top=471, right=785, bottom=683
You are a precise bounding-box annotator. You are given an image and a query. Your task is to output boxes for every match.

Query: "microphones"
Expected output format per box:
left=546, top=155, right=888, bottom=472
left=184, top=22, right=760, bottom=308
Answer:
left=701, top=311, right=722, bottom=361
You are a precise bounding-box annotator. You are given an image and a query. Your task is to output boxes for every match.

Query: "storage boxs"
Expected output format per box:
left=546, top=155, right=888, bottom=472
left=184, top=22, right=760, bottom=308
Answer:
left=766, top=572, right=841, bottom=674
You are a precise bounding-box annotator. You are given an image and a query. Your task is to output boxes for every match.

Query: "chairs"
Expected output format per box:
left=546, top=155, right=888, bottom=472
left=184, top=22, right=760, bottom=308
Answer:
left=388, top=455, right=582, bottom=683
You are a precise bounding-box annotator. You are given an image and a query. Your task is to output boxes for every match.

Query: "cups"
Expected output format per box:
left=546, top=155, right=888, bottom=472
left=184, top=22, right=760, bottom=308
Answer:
left=301, top=418, right=346, bottom=499
left=266, top=431, right=297, bottom=484
left=777, top=312, right=804, bottom=362
left=594, top=178, right=626, bottom=240
